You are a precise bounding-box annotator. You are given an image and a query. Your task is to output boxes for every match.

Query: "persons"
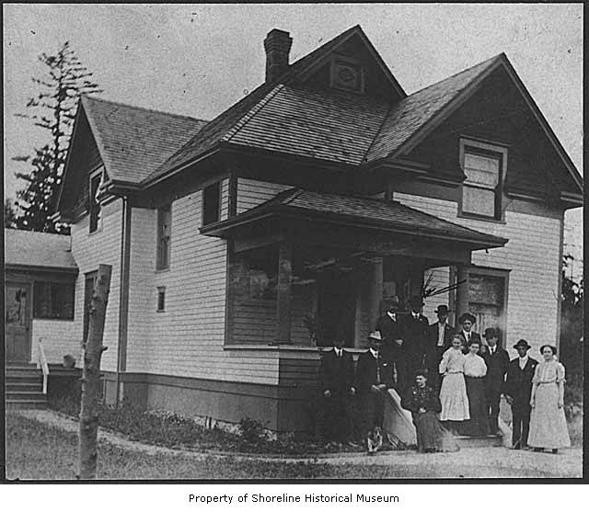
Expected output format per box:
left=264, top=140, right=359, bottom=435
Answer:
left=464, top=339, right=490, bottom=437
left=378, top=295, right=482, bottom=397
left=320, top=334, right=359, bottom=448
left=527, top=345, right=571, bottom=454
left=400, top=367, right=460, bottom=452
left=357, top=331, right=395, bottom=444
left=504, top=339, right=539, bottom=450
left=437, top=334, right=470, bottom=436
left=481, top=328, right=510, bottom=436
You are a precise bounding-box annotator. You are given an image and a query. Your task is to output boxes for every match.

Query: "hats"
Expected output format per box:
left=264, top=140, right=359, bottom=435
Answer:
left=408, top=295, right=425, bottom=306
left=384, top=296, right=399, bottom=304
left=482, top=328, right=498, bottom=337
left=459, top=313, right=476, bottom=324
left=513, top=339, right=531, bottom=350
left=434, top=305, right=451, bottom=313
left=368, top=331, right=381, bottom=340
